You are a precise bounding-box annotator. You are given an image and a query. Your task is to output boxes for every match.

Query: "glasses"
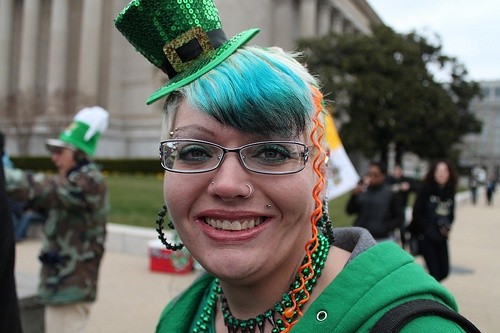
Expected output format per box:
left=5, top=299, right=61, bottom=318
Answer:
left=157, top=139, right=313, bottom=175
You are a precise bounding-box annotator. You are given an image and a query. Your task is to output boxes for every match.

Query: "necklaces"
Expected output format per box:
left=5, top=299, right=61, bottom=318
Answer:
left=192, top=232, right=329, bottom=333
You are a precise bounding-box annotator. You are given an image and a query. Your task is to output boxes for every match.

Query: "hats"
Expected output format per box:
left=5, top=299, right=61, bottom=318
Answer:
left=46, top=103, right=110, bottom=157
left=112, top=0, right=262, bottom=106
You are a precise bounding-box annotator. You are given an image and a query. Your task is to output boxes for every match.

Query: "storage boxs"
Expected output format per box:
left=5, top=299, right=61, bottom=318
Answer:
left=150, top=247, right=194, bottom=274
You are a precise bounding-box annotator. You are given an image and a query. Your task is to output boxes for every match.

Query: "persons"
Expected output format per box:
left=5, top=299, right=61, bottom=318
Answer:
left=155, top=46, right=481, bottom=333
left=4, top=106, right=110, bottom=333
left=464, top=161, right=496, bottom=206
